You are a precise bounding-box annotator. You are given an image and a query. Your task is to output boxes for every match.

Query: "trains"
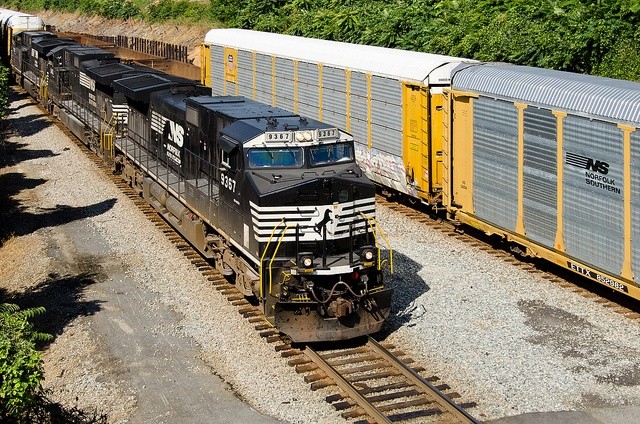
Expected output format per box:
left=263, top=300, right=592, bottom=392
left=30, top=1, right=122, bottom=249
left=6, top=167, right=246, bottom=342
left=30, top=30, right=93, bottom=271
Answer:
left=197, top=26, right=639, bottom=302
left=0, top=6, right=394, bottom=341
